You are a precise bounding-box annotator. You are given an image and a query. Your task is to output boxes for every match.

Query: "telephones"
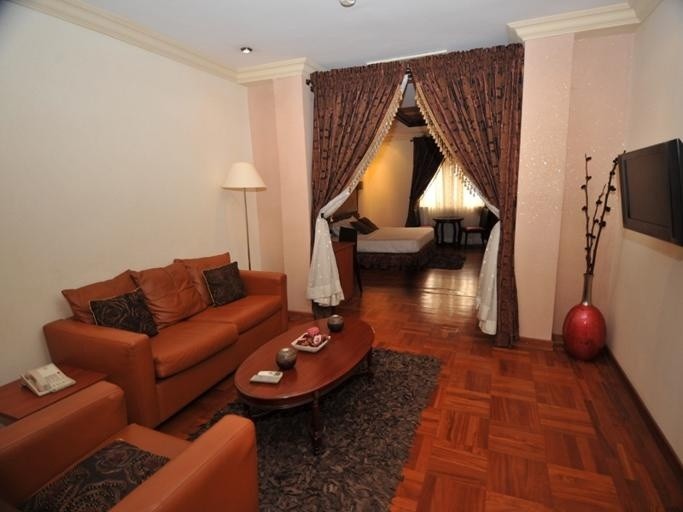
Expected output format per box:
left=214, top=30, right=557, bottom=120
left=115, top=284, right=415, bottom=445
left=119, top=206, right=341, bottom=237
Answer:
left=19, top=362, right=77, bottom=397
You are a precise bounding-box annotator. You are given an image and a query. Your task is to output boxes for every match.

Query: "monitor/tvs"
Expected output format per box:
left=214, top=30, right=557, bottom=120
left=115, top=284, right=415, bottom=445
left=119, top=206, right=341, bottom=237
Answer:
left=617, top=138, right=683, bottom=248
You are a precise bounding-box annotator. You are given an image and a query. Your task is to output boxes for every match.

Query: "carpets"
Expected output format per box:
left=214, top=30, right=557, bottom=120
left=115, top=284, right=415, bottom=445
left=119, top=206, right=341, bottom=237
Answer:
left=426, top=253, right=465, bottom=270
left=186, top=347, right=444, bottom=512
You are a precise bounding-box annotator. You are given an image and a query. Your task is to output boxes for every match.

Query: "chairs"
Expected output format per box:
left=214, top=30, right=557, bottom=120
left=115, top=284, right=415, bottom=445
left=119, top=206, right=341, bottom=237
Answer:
left=0, top=380, right=260, bottom=512
left=339, top=226, right=363, bottom=293
left=462, top=207, right=489, bottom=249
left=412, top=207, right=434, bottom=228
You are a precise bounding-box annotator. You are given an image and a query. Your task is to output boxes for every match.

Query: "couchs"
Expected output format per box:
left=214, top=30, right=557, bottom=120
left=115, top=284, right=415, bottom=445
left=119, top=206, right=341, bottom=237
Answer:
left=43, top=251, right=289, bottom=428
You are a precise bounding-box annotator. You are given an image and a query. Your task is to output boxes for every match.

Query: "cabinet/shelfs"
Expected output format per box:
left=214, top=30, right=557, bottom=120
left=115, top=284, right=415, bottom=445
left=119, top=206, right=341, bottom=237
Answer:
left=332, top=241, right=355, bottom=304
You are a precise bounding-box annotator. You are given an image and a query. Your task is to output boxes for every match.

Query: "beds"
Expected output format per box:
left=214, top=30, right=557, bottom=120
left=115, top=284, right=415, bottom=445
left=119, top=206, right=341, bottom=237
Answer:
left=326, top=181, right=435, bottom=276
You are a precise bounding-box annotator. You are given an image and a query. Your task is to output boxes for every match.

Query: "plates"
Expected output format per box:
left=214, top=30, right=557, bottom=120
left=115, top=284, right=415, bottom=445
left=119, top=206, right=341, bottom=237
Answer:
left=290, top=331, right=332, bottom=353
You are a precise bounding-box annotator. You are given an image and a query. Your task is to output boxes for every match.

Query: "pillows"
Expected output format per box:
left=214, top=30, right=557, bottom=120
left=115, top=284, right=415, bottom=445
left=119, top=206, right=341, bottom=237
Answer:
left=330, top=215, right=360, bottom=237
left=130, top=261, right=208, bottom=330
left=201, top=261, right=247, bottom=307
left=88, top=287, right=160, bottom=336
left=350, top=216, right=379, bottom=234
left=61, top=270, right=137, bottom=325
left=173, top=252, right=231, bottom=306
left=14, top=438, right=170, bottom=512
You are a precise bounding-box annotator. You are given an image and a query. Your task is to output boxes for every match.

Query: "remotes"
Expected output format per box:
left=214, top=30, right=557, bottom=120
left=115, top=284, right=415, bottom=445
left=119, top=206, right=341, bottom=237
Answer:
left=249, top=375, right=282, bottom=384
left=257, top=370, right=284, bottom=378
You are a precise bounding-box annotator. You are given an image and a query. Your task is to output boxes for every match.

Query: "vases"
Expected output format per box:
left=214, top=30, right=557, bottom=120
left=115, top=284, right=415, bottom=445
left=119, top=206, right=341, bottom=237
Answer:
left=563, top=273, right=608, bottom=360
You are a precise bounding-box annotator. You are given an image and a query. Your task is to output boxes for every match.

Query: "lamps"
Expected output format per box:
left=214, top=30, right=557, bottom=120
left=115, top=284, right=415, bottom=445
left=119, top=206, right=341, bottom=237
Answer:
left=220, top=162, right=268, bottom=270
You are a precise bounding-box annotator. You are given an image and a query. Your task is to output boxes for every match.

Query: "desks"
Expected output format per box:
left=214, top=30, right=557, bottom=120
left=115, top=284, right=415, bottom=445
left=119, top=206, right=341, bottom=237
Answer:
left=432, top=216, right=464, bottom=249
